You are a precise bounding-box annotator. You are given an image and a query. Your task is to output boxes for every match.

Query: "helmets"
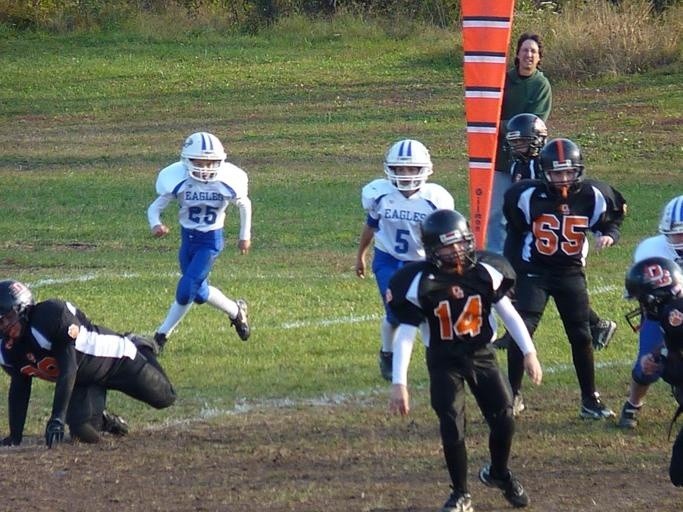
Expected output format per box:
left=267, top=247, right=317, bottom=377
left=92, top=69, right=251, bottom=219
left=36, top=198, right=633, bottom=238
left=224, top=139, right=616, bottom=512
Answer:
left=625, top=256, right=682, bottom=321
left=540, top=138, right=583, bottom=199
left=424, top=209, right=475, bottom=268
left=385, top=140, right=434, bottom=192
left=181, top=132, right=228, bottom=182
left=506, top=113, right=548, bottom=158
left=0, top=279, right=33, bottom=321
left=658, top=195, right=683, bottom=258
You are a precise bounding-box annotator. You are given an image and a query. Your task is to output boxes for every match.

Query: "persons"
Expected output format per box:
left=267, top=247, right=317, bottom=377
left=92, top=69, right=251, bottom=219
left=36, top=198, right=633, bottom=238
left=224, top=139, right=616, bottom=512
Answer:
left=492, top=112, right=618, bottom=351
left=618, top=193, right=683, bottom=428
left=482, top=30, right=553, bottom=255
left=0, top=279, right=178, bottom=451
left=622, top=256, right=683, bottom=487
left=387, top=209, right=543, bottom=512
left=147, top=130, right=252, bottom=355
left=503, top=137, right=617, bottom=420
left=355, top=139, right=455, bottom=381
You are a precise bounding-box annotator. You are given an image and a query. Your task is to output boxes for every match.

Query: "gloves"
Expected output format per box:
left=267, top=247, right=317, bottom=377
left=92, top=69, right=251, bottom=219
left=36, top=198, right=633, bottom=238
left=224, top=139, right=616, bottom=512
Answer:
left=44, top=416, right=66, bottom=450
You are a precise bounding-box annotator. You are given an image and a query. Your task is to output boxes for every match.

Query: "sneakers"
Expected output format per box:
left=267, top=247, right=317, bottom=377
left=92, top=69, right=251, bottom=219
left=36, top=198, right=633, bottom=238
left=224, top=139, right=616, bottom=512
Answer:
left=227, top=297, right=251, bottom=343
left=377, top=344, right=395, bottom=383
left=100, top=407, right=132, bottom=438
left=124, top=331, right=169, bottom=355
left=438, top=484, right=473, bottom=512
left=478, top=462, right=530, bottom=508
left=619, top=398, right=644, bottom=428
left=591, top=318, right=618, bottom=352
left=579, top=391, right=616, bottom=420
